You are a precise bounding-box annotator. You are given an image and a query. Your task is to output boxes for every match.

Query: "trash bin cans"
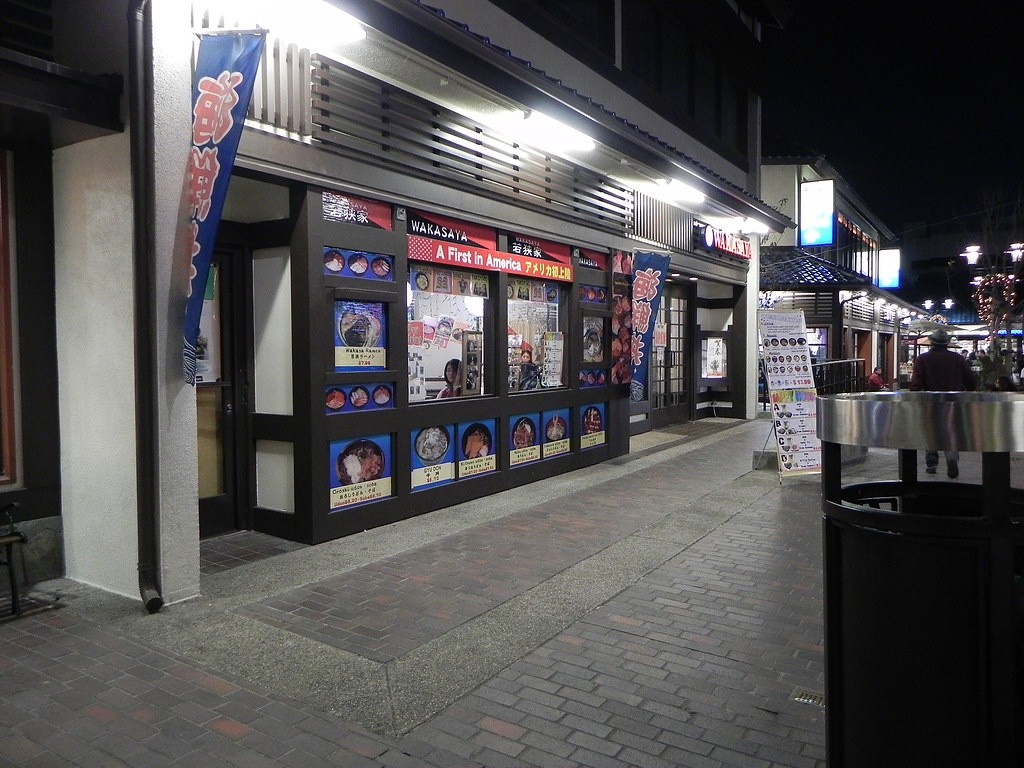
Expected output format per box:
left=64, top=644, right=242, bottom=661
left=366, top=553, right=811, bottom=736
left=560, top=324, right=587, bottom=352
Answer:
left=814, top=389, right=1024, bottom=768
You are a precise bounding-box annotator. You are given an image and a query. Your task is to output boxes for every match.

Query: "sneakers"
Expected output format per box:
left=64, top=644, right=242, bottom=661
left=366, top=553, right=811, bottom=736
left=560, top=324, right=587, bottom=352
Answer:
left=947, top=459, right=959, bottom=478
left=925, top=466, right=937, bottom=473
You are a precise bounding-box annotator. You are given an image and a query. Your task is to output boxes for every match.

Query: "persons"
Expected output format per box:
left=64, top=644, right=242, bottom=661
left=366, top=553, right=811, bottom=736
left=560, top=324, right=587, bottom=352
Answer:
left=962, top=344, right=1024, bottom=385
left=869, top=367, right=889, bottom=393
left=517, top=350, right=532, bottom=367
left=435, top=359, right=463, bottom=399
left=909, top=329, right=974, bottom=479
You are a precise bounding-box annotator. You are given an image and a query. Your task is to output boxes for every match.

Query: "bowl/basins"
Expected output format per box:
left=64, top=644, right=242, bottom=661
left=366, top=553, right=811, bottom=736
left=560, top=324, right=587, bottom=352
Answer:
left=335, top=406, right=605, bottom=487
left=338, top=304, right=382, bottom=350
left=414, top=271, right=430, bottom=291
left=587, top=371, right=596, bottom=385
left=348, top=385, right=370, bottom=407
left=370, top=256, right=392, bottom=277
left=765, top=337, right=810, bottom=375
left=596, top=289, right=605, bottom=302
left=348, top=253, right=368, bottom=276
left=372, top=384, right=391, bottom=404
left=324, top=388, right=348, bottom=411
left=578, top=285, right=586, bottom=300
left=596, top=371, right=606, bottom=385
left=587, top=287, right=596, bottom=301
left=322, top=250, right=345, bottom=273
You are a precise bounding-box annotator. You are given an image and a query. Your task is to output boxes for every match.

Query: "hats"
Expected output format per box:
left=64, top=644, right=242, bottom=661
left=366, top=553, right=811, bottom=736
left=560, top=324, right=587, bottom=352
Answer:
left=927, top=329, right=950, bottom=344
